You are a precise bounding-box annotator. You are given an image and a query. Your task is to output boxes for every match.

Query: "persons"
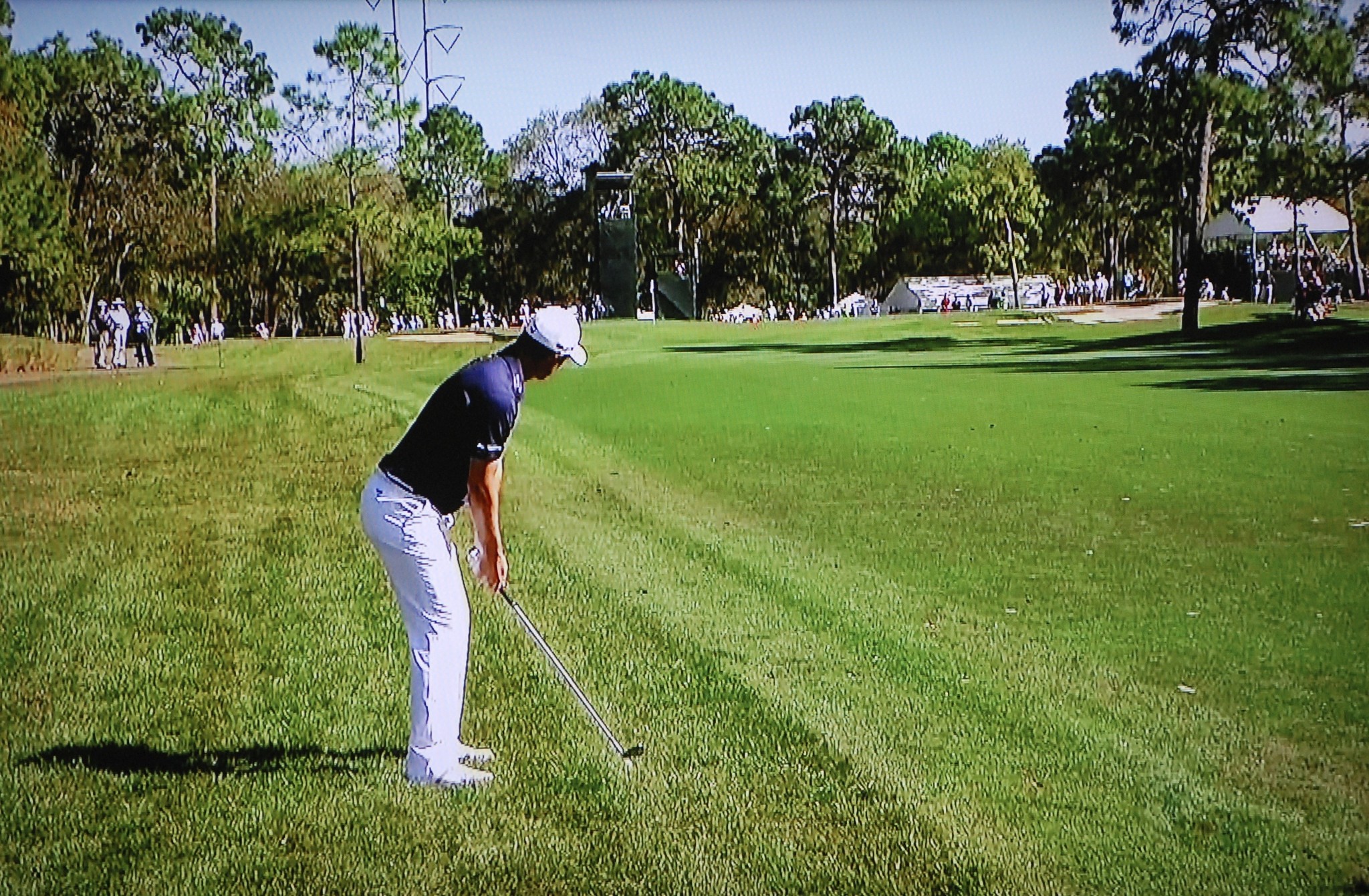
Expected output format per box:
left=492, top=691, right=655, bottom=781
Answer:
left=1172, top=242, right=1369, bottom=323
left=354, top=304, right=593, bottom=790
left=90, top=296, right=271, bottom=371
left=337, top=291, right=617, bottom=343
left=700, top=261, right=1148, bottom=327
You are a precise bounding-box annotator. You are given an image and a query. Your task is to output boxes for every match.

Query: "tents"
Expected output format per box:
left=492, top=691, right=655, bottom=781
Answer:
left=1199, top=193, right=1357, bottom=296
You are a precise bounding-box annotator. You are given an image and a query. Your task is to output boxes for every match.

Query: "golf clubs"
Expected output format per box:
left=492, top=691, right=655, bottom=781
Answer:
left=464, top=545, right=645, bottom=758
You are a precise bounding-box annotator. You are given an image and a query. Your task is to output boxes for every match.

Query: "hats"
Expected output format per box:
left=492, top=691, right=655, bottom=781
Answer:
left=526, top=305, right=587, bottom=366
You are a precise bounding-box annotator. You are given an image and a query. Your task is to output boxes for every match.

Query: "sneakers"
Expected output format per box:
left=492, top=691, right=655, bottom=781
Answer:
left=454, top=740, right=492, bottom=760
left=407, top=763, right=493, bottom=784
